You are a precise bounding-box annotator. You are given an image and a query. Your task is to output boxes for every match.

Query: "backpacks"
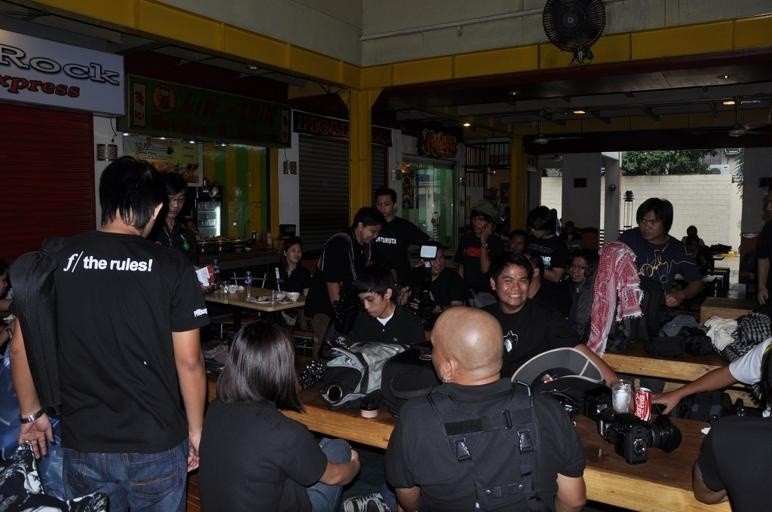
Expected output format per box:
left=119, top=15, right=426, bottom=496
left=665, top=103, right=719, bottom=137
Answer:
left=379, top=340, right=443, bottom=421
left=320, top=337, right=413, bottom=412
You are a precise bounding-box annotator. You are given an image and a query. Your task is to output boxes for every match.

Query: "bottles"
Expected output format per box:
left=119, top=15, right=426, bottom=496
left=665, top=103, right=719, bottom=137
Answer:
left=210, top=258, right=222, bottom=288
left=198, top=202, right=223, bottom=238
left=251, top=230, right=273, bottom=250
left=246, top=271, right=255, bottom=288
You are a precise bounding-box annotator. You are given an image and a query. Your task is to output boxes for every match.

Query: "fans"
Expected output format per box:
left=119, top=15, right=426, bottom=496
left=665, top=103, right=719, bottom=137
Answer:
left=543, top=0, right=607, bottom=62
left=501, top=110, right=579, bottom=148
left=688, top=97, right=771, bottom=143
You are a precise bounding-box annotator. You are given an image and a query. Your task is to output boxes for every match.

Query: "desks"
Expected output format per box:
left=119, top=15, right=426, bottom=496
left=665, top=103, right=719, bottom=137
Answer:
left=277, top=266, right=771, bottom=512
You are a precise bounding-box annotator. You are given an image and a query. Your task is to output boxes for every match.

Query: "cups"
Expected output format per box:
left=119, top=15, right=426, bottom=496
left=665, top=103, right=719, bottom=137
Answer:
left=610, top=380, right=636, bottom=416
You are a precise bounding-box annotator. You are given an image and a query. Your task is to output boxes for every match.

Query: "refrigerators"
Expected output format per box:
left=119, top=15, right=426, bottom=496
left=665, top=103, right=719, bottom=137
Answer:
left=191, top=187, right=223, bottom=243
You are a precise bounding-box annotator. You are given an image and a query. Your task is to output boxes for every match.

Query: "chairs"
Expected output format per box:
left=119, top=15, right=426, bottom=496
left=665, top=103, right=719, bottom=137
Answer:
left=195, top=272, right=314, bottom=397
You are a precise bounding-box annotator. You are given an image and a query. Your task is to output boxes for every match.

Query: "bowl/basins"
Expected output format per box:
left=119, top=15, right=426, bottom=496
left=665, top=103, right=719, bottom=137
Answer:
left=221, top=285, right=245, bottom=296
left=235, top=244, right=253, bottom=254
left=273, top=291, right=299, bottom=301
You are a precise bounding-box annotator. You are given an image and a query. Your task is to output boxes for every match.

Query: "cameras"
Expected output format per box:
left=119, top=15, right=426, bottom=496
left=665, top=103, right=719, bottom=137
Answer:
left=594, top=409, right=681, bottom=465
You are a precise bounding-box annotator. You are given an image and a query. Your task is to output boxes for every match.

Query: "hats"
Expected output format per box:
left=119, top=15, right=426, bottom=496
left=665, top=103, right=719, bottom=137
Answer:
left=470, top=198, right=500, bottom=223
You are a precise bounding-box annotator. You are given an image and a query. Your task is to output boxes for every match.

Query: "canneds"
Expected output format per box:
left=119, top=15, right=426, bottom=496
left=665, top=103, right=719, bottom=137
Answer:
left=634, top=387, right=652, bottom=423
